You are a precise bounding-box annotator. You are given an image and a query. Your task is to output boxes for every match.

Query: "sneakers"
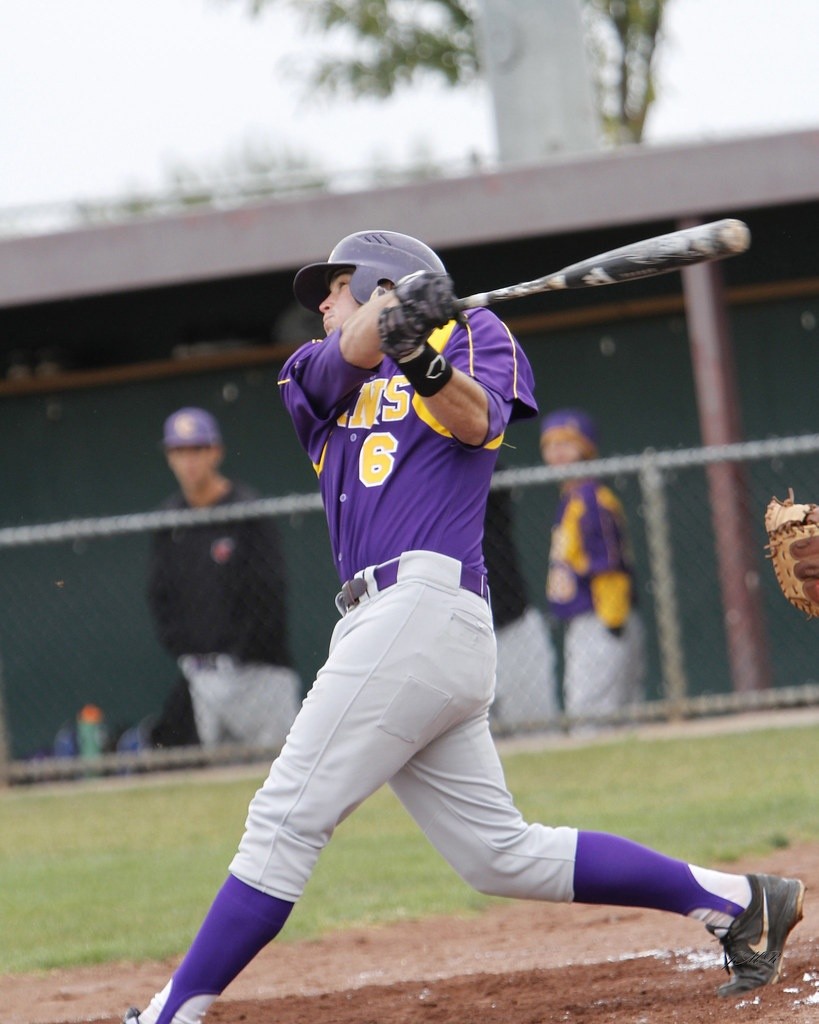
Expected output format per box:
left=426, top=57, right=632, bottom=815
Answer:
left=718, top=875, right=804, bottom=997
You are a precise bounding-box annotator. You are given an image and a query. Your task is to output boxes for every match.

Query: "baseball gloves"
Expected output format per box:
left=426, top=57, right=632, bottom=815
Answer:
left=760, top=486, right=819, bottom=622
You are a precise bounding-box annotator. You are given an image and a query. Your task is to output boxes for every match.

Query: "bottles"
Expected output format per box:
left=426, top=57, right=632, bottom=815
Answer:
left=78, top=705, right=102, bottom=758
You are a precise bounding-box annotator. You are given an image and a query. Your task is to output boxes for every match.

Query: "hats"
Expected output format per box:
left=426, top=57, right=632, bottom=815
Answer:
left=163, top=410, right=218, bottom=450
left=540, top=409, right=598, bottom=452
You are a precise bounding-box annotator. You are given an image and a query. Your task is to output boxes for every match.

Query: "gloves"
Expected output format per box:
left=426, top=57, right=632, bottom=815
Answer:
left=392, top=269, right=459, bottom=332
left=377, top=301, right=435, bottom=360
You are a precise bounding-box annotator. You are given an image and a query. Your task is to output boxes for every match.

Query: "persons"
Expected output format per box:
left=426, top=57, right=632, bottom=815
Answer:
left=482, top=408, right=646, bottom=741
left=145, top=406, right=301, bottom=746
left=764, top=488, right=819, bottom=618
left=118, top=231, right=805, bottom=1024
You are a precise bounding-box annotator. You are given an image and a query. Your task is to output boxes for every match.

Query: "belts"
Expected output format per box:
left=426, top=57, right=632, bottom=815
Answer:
left=335, top=560, right=486, bottom=614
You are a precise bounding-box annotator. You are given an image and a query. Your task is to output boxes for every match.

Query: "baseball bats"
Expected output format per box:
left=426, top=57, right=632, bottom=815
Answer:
left=456, top=218, right=752, bottom=313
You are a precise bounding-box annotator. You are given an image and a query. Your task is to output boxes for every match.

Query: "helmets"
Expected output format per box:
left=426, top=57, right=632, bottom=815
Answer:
left=292, top=230, right=447, bottom=311
